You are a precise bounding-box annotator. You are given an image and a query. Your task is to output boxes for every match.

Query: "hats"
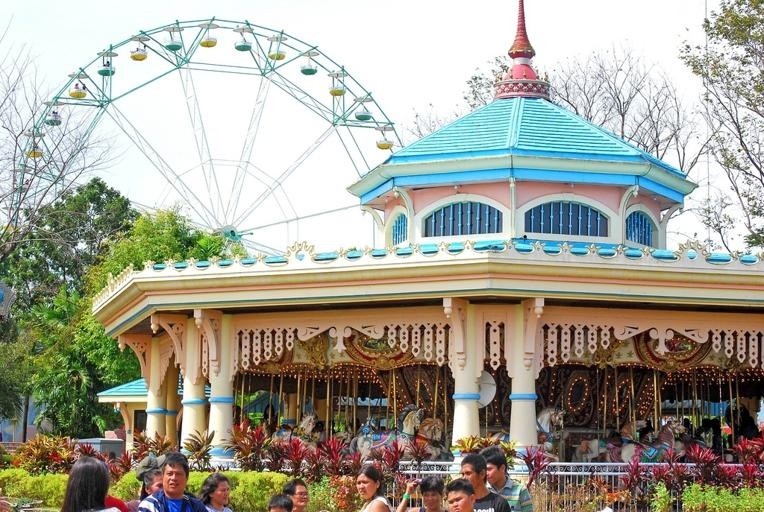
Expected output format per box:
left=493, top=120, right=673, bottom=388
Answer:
left=137, top=453, right=166, bottom=481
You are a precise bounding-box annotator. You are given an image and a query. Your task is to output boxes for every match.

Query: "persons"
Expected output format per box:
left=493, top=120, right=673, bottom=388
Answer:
left=356, top=464, right=392, bottom=512
left=58, top=445, right=233, bottom=512
left=397, top=446, right=533, bottom=512
left=267, top=479, right=309, bottom=512
